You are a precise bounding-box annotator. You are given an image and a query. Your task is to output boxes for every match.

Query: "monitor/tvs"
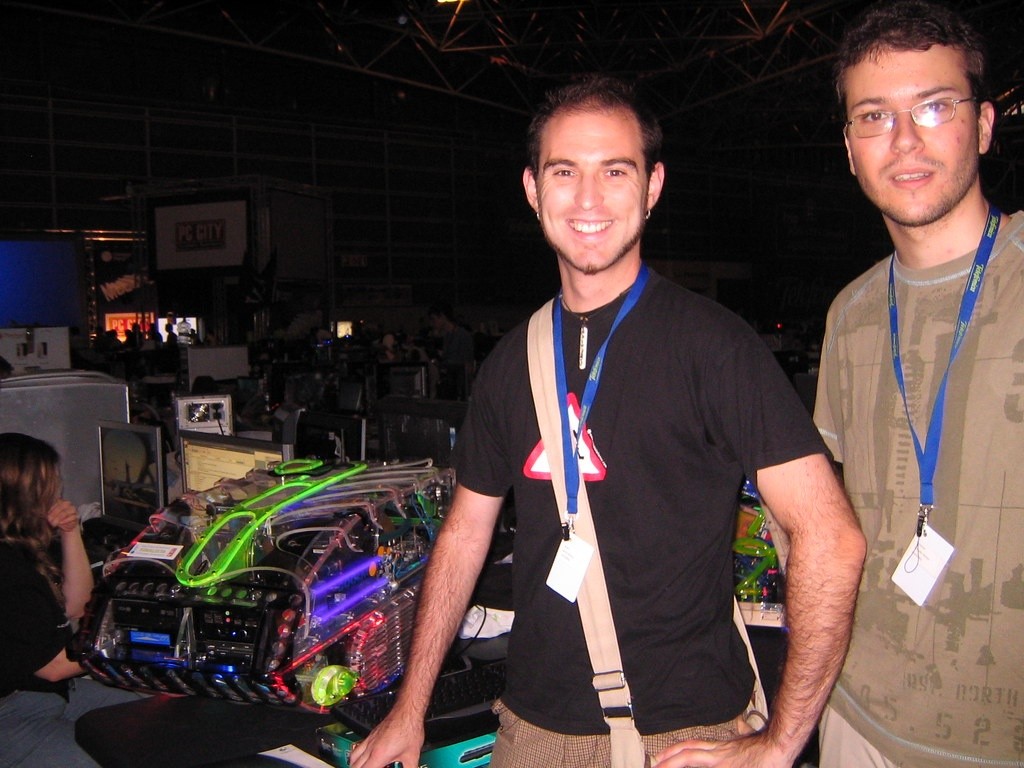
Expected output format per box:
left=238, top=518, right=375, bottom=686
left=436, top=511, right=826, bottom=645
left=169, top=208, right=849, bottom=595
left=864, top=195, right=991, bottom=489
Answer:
left=294, top=410, right=366, bottom=463
left=98, top=420, right=168, bottom=549
left=178, top=430, right=295, bottom=495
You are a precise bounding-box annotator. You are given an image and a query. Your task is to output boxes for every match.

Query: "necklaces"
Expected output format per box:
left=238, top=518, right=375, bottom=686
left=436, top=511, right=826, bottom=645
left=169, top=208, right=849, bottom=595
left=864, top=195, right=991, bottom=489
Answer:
left=557, top=283, right=630, bottom=370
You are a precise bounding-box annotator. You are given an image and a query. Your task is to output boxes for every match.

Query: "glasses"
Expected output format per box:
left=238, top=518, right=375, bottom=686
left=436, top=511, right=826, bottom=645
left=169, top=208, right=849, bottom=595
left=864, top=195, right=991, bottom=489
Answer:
left=845, top=96, right=977, bottom=139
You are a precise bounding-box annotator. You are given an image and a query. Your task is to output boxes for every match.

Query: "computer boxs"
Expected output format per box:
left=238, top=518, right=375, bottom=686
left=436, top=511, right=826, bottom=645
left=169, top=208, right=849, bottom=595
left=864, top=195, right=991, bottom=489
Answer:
left=0, top=368, right=130, bottom=515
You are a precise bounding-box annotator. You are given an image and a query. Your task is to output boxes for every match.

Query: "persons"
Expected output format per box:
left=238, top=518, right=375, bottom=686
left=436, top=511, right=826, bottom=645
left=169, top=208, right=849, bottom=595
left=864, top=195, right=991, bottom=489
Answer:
left=382, top=333, right=398, bottom=364
left=0, top=431, right=155, bottom=768
left=426, top=300, right=474, bottom=401
left=90, top=322, right=178, bottom=376
left=809, top=0, right=1024, bottom=768
left=344, top=69, right=870, bottom=768
